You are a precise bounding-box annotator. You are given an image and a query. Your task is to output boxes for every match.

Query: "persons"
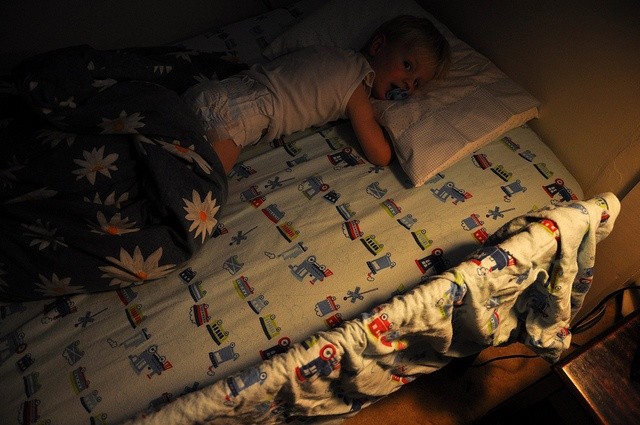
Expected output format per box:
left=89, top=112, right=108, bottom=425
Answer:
left=182, top=15, right=453, bottom=173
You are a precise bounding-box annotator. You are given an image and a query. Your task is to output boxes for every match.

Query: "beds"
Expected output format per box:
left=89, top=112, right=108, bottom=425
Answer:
left=1, top=1, right=640, bottom=424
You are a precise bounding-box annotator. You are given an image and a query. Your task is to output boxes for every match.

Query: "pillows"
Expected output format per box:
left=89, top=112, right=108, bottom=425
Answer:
left=261, top=0, right=544, bottom=190
